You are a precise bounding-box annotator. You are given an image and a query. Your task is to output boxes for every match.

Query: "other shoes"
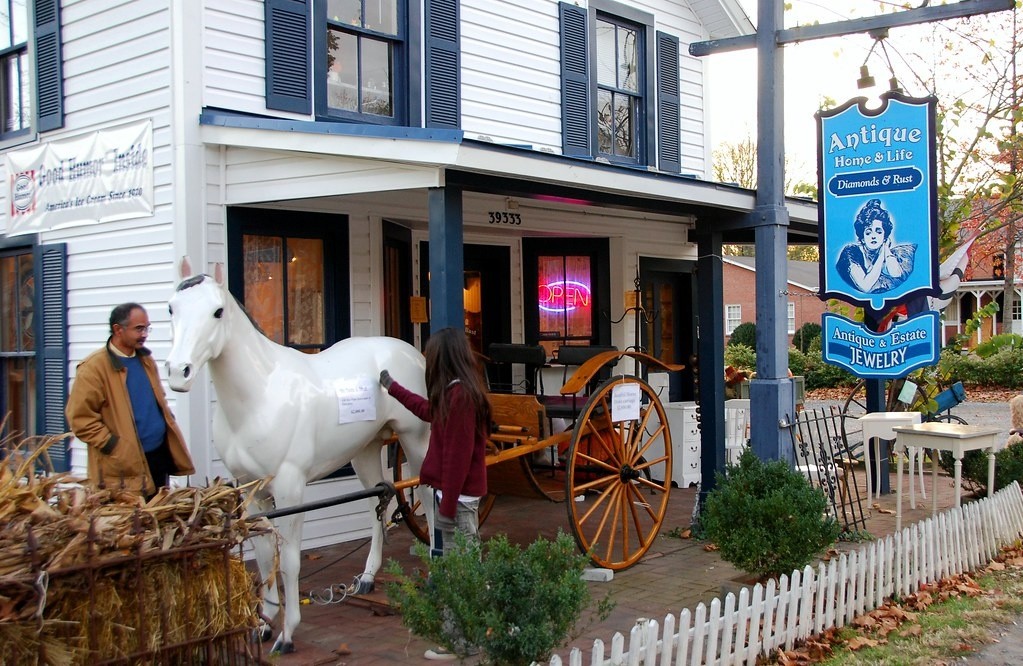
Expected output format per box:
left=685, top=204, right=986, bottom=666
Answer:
left=424, top=647, right=458, bottom=661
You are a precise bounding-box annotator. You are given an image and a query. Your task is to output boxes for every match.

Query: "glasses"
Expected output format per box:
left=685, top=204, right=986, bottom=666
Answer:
left=121, top=325, right=152, bottom=333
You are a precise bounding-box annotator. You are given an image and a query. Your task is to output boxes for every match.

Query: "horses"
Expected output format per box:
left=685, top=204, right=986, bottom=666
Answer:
left=164, top=255, right=436, bottom=658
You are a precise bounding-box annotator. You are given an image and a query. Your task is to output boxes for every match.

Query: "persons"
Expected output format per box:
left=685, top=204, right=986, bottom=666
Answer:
left=379, top=327, right=487, bottom=660
left=65, top=302, right=195, bottom=498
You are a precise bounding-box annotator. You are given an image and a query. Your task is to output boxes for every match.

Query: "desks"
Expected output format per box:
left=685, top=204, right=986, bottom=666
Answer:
left=892, top=422, right=1002, bottom=533
left=859, top=412, right=928, bottom=509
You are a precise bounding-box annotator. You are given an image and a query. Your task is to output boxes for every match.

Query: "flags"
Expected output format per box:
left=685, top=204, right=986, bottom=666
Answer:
left=878, top=224, right=982, bottom=333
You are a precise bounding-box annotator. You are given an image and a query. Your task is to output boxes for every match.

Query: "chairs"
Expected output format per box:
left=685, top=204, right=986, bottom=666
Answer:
left=725, top=399, right=750, bottom=476
left=535, top=345, right=619, bottom=498
left=484, top=342, right=546, bottom=450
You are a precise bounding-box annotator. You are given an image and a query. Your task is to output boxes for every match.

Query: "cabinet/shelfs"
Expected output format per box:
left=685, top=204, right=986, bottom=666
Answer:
left=726, top=408, right=745, bottom=465
left=641, top=401, right=701, bottom=488
